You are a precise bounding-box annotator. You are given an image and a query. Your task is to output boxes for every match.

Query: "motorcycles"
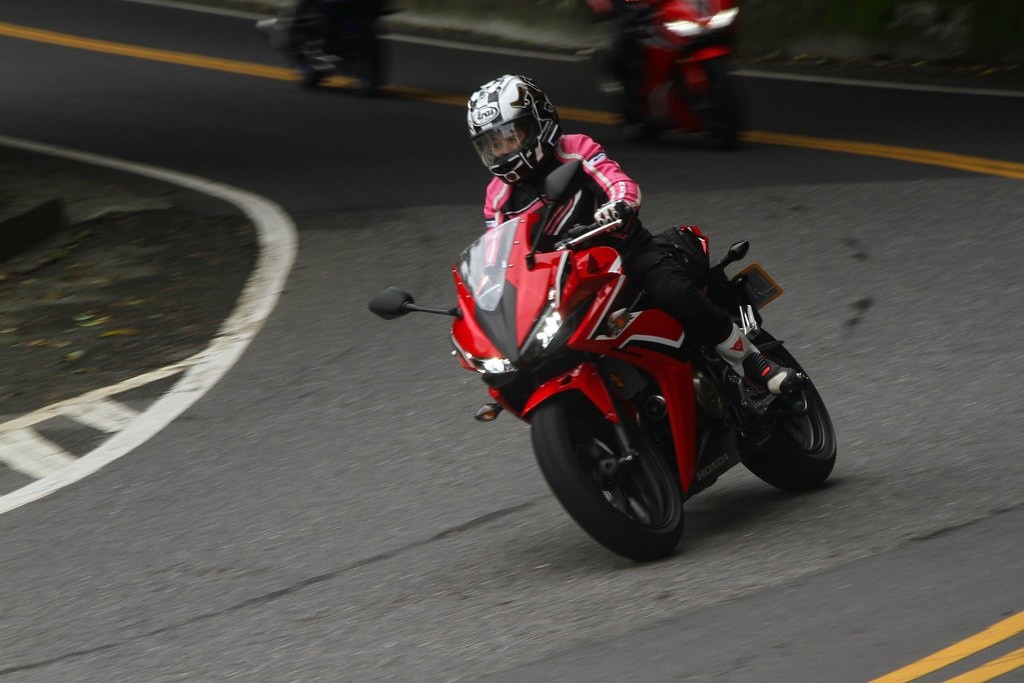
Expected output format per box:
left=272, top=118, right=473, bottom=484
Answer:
left=367, top=159, right=839, bottom=563
left=574, top=0, right=745, bottom=155
left=256, top=0, right=411, bottom=101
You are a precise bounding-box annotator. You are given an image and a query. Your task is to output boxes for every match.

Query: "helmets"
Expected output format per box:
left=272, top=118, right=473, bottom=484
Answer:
left=466, top=74, right=563, bottom=187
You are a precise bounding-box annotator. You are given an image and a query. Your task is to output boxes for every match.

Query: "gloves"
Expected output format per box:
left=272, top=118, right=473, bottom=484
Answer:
left=593, top=200, right=628, bottom=233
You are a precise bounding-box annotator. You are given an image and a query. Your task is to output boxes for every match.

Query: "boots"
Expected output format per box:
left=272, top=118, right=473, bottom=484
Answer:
left=715, top=323, right=798, bottom=397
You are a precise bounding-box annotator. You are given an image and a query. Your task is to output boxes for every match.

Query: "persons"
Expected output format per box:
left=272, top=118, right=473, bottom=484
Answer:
left=274, top=0, right=396, bottom=82
left=467, top=75, right=806, bottom=402
left=590, top=0, right=710, bottom=115
